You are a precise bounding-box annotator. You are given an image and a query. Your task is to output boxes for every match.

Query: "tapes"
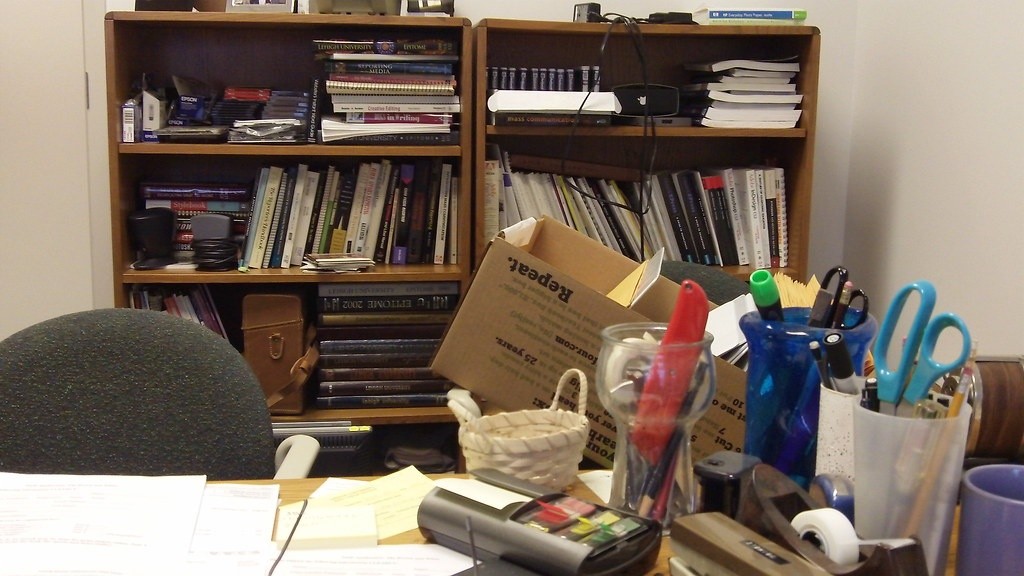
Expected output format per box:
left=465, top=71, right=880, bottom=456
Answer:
left=791, top=507, right=861, bottom=567
left=607, top=332, right=657, bottom=405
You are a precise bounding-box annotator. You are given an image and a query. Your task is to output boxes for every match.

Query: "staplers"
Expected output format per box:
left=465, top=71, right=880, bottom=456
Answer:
left=666, top=510, right=833, bottom=576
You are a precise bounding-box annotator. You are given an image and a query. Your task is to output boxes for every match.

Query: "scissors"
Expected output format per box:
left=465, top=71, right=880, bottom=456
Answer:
left=818, top=265, right=870, bottom=329
left=875, top=284, right=971, bottom=417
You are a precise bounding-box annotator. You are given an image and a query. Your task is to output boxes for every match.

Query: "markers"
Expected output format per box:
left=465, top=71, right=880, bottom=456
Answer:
left=745, top=268, right=785, bottom=322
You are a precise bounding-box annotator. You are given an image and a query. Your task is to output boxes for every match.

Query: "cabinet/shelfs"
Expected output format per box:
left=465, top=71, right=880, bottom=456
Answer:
left=473, top=17, right=823, bottom=287
left=102, top=10, right=472, bottom=478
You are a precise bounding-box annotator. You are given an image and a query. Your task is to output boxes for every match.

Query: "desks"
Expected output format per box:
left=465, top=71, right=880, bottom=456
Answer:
left=0, top=473, right=676, bottom=576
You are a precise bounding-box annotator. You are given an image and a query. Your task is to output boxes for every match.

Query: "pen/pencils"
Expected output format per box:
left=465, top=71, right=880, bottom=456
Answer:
left=833, top=278, right=852, bottom=328
left=639, top=362, right=712, bottom=517
left=808, top=333, right=859, bottom=391
left=862, top=336, right=973, bottom=576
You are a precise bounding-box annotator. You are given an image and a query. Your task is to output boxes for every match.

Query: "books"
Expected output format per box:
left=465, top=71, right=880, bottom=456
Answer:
left=121, top=283, right=463, bottom=473
left=489, top=152, right=791, bottom=270
left=309, top=39, right=462, bottom=145
left=121, top=158, right=461, bottom=272
left=694, top=8, right=808, bottom=128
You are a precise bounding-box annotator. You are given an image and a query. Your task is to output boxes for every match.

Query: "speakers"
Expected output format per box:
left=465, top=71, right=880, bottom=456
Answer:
left=191, top=214, right=236, bottom=271
left=128, top=206, right=181, bottom=269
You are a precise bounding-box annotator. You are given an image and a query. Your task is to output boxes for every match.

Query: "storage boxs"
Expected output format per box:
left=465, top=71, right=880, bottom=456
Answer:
left=428, top=215, right=750, bottom=470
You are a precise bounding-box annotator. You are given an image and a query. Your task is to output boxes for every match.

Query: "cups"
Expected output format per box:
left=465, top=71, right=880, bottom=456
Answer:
left=594, top=323, right=718, bottom=538
left=739, top=307, right=878, bottom=494
left=853, top=394, right=973, bottom=576
left=814, top=377, right=878, bottom=497
left=956, top=463, right=1024, bottom=576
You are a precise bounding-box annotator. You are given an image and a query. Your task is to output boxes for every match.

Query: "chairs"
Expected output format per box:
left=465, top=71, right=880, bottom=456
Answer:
left=1, top=307, right=321, bottom=480
